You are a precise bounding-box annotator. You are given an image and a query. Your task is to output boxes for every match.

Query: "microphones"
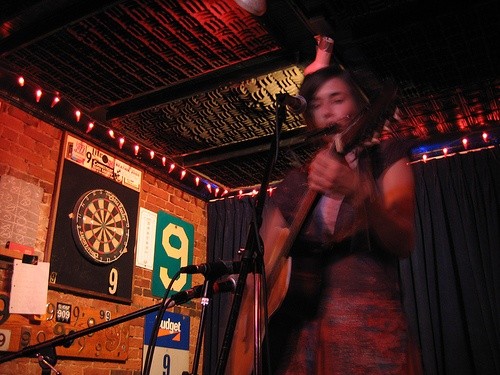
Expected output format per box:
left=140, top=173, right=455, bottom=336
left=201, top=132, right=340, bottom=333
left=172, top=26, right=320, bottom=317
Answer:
left=169, top=274, right=239, bottom=301
left=180, top=260, right=253, bottom=276
left=275, top=93, right=308, bottom=115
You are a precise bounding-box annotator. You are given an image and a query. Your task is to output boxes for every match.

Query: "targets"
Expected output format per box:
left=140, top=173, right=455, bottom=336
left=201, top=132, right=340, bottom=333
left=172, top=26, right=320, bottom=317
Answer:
left=69, top=189, right=129, bottom=264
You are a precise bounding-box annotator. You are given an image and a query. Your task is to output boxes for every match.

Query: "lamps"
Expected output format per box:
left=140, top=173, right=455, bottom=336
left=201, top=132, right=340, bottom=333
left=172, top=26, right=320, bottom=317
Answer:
left=235, top=0, right=266, bottom=16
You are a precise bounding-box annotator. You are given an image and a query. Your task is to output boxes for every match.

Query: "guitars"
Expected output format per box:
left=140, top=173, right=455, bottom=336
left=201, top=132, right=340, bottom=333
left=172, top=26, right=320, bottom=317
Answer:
left=205, top=83, right=401, bottom=375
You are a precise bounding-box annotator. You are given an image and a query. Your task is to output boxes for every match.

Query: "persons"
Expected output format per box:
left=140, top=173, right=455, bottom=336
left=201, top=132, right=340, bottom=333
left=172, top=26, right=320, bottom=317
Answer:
left=250, top=65, right=422, bottom=375
